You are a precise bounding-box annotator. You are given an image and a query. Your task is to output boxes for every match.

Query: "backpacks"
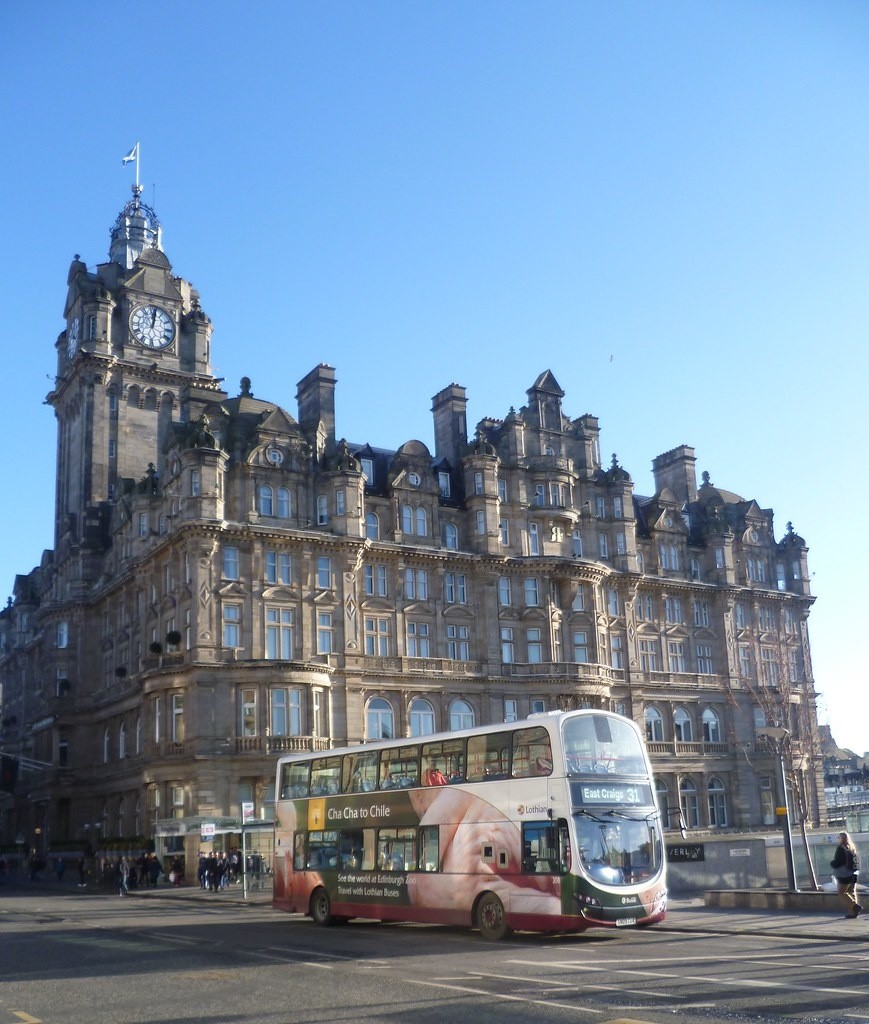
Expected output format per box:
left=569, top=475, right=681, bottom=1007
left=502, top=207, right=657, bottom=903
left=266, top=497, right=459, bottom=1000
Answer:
left=847, top=850, right=861, bottom=871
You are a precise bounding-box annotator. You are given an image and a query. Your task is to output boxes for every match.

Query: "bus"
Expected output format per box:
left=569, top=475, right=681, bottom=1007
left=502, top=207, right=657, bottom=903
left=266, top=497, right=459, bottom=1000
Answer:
left=270, top=706, right=690, bottom=945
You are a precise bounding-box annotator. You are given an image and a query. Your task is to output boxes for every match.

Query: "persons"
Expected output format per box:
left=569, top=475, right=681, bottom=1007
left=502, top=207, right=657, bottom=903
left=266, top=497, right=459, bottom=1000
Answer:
left=384, top=860, right=394, bottom=871
left=0, top=845, right=185, bottom=897
left=346, top=857, right=359, bottom=871
left=430, top=760, right=447, bottom=785
left=197, top=846, right=266, bottom=894
left=377, top=852, right=387, bottom=870
left=830, top=831, right=863, bottom=919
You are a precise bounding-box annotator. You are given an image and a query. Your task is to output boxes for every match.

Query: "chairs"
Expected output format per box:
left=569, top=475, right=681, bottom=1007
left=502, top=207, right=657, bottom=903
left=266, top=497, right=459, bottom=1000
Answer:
left=282, top=766, right=607, bottom=800
left=293, top=847, right=403, bottom=870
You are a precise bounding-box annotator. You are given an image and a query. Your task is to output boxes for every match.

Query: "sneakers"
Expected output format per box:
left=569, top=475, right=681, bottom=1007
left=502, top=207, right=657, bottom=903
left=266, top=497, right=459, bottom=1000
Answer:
left=845, top=905, right=863, bottom=918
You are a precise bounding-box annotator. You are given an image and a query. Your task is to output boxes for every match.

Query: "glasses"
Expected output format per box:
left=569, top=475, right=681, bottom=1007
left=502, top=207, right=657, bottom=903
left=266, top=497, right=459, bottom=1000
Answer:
left=838, top=837, right=842, bottom=839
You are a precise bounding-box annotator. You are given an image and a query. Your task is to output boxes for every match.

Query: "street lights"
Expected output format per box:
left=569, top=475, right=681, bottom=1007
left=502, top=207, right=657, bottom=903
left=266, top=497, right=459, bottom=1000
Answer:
left=755, top=720, right=801, bottom=890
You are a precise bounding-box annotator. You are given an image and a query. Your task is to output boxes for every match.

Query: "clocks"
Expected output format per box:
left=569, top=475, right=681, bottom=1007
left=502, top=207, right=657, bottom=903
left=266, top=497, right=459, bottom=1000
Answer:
left=129, top=303, right=177, bottom=351
